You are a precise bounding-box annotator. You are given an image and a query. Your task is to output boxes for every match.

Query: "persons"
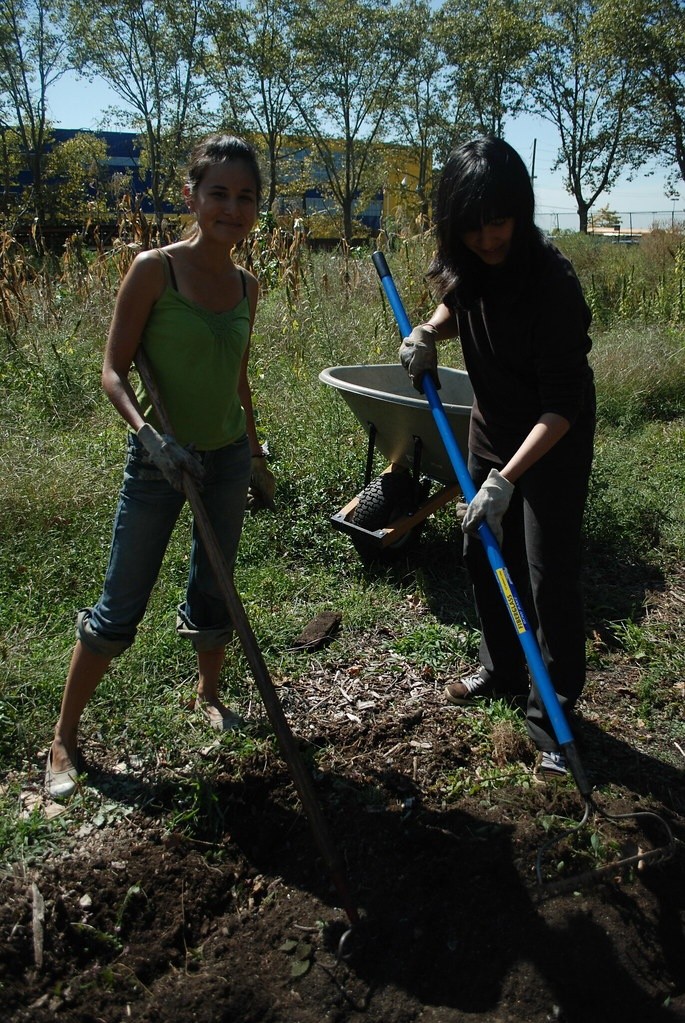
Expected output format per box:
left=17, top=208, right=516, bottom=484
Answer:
left=43, top=134, right=277, bottom=801
left=398, top=136, right=597, bottom=751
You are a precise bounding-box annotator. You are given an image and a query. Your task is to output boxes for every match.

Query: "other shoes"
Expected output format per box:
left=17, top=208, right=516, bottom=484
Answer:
left=45, top=746, right=79, bottom=798
left=194, top=700, right=242, bottom=732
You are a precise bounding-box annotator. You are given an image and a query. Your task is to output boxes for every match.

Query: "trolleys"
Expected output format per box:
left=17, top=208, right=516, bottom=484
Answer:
left=319, top=364, right=473, bottom=569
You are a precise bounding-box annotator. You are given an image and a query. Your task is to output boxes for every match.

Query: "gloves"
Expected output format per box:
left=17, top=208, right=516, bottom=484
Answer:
left=456, top=468, right=515, bottom=543
left=245, top=454, right=277, bottom=517
left=138, top=422, right=206, bottom=492
left=399, top=326, right=441, bottom=395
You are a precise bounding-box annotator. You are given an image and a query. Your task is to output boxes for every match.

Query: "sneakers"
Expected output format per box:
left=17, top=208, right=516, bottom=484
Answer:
left=533, top=748, right=568, bottom=782
left=444, top=673, right=500, bottom=705
